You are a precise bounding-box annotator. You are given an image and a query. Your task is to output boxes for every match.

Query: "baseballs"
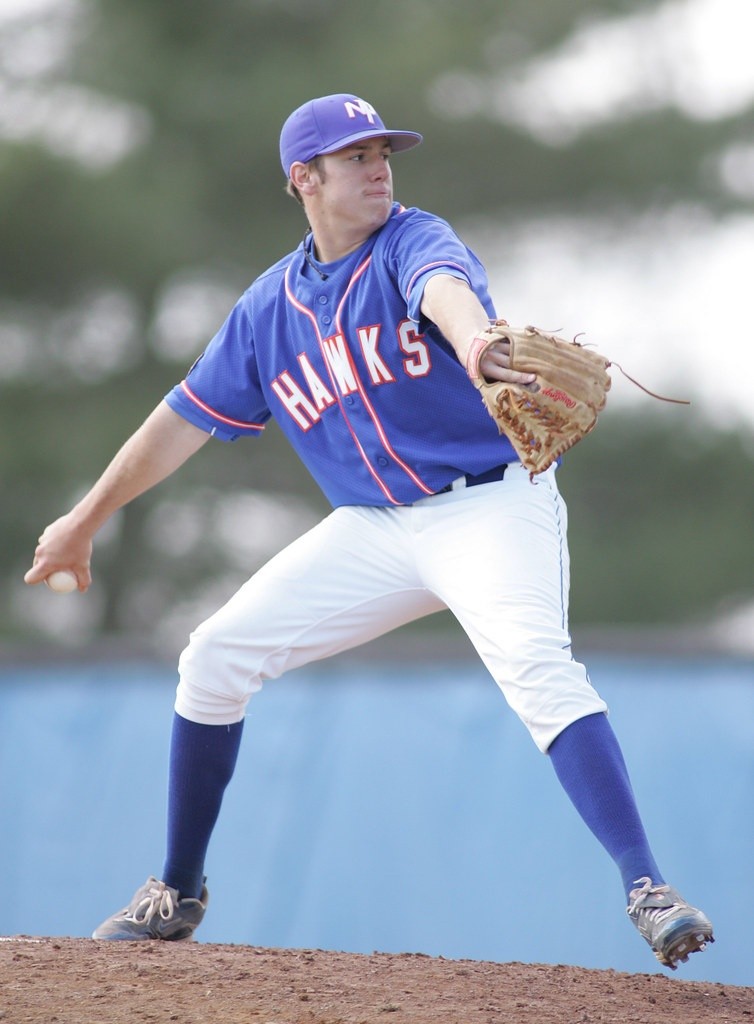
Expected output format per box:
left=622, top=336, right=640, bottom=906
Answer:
left=44, top=568, right=78, bottom=593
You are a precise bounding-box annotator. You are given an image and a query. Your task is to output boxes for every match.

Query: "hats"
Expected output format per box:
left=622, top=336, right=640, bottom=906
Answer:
left=280, top=94, right=423, bottom=178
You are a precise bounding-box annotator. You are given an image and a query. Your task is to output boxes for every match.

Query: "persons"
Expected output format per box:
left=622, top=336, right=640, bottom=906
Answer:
left=23, top=94, right=716, bottom=971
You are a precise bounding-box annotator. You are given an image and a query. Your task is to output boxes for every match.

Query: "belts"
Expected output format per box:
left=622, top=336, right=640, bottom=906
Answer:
left=435, top=462, right=508, bottom=494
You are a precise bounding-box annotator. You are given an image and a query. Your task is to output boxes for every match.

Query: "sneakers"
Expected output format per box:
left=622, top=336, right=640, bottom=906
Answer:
left=95, top=876, right=210, bottom=941
left=626, top=877, right=715, bottom=970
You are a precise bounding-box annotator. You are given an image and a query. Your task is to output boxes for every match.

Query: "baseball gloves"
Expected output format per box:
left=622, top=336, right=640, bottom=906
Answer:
left=467, top=319, right=612, bottom=485
